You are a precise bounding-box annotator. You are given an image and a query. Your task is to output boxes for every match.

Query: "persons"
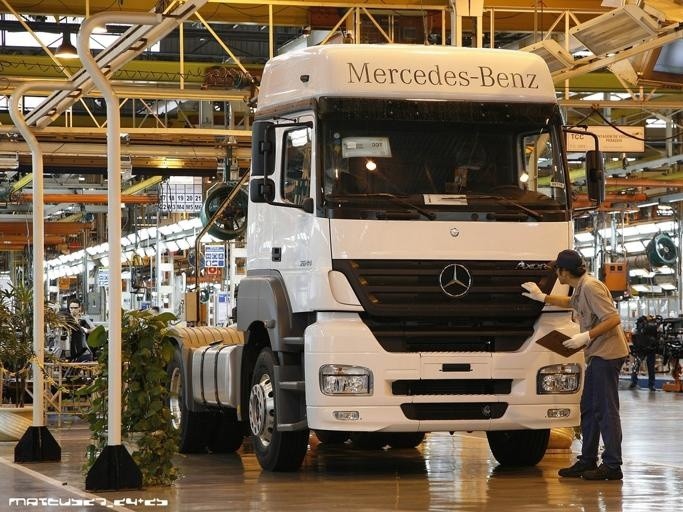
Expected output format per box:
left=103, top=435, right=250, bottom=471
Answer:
left=624, top=315, right=659, bottom=392
left=59, top=299, right=96, bottom=362
left=518, top=250, right=630, bottom=482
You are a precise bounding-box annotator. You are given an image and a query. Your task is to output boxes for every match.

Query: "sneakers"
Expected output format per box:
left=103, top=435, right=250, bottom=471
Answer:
left=582, top=463, right=623, bottom=480
left=649, top=386, right=657, bottom=391
left=630, top=382, right=636, bottom=388
left=558, top=461, right=597, bottom=478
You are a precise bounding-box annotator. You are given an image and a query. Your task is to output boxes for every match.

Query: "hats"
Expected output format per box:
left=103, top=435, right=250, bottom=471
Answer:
left=547, top=249, right=585, bottom=271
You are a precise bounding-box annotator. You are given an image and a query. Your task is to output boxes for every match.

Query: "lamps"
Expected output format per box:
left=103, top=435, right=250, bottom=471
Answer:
left=53, top=30, right=79, bottom=59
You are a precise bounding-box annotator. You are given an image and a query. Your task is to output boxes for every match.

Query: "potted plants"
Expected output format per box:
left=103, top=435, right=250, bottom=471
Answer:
left=80, top=307, right=182, bottom=487
left=0, top=271, right=72, bottom=441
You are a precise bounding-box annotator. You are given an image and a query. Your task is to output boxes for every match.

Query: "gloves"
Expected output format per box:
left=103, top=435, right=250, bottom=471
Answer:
left=521, top=282, right=547, bottom=303
left=563, top=331, right=592, bottom=349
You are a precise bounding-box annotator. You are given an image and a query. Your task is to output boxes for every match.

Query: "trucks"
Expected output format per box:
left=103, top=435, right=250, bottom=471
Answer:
left=152, top=42, right=606, bottom=474
